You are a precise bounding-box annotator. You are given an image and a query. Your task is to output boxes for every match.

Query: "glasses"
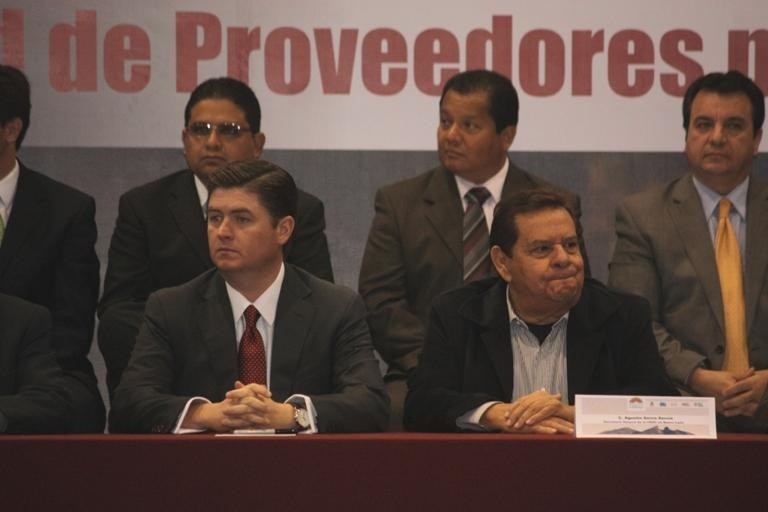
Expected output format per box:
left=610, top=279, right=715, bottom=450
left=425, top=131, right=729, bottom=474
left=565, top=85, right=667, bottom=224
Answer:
left=185, top=120, right=251, bottom=138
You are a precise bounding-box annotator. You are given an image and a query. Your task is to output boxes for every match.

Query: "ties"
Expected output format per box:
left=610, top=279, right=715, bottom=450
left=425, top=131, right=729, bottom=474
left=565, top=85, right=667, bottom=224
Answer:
left=712, top=197, right=750, bottom=373
left=459, top=187, right=493, bottom=283
left=237, top=305, right=267, bottom=393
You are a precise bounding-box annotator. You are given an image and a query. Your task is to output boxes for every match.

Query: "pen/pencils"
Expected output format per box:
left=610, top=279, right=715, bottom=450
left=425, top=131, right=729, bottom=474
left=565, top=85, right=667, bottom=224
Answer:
left=233, top=429, right=297, bottom=434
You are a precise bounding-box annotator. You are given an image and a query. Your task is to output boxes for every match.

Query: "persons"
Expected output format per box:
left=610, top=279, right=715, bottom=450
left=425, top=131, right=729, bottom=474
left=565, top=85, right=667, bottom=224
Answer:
left=94, top=75, right=336, bottom=400
left=0, top=290, right=97, bottom=434
left=108, top=158, right=394, bottom=434
left=606, top=69, right=767, bottom=432
left=0, top=64, right=107, bottom=432
left=355, top=68, right=592, bottom=434
left=401, top=189, right=662, bottom=436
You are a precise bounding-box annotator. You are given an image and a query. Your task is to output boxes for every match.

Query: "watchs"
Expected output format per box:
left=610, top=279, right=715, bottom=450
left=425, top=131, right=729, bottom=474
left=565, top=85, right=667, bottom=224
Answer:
left=289, top=401, right=310, bottom=433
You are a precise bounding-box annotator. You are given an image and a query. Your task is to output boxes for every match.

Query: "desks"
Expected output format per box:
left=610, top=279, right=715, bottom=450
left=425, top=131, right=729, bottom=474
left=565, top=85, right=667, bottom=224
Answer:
left=0, top=432, right=768, bottom=512
